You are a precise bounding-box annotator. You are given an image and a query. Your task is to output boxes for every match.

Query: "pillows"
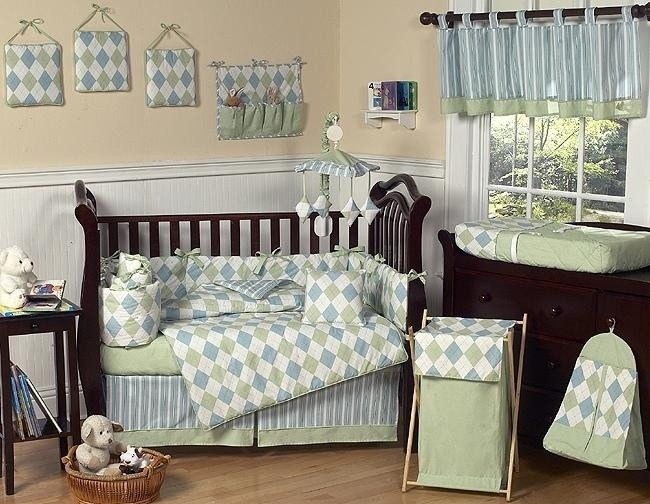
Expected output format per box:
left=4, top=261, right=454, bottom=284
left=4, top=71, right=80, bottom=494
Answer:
left=301, top=268, right=367, bottom=327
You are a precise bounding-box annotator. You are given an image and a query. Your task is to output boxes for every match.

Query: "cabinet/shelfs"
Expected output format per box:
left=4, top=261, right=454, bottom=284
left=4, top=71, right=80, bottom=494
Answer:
left=438, top=221, right=650, bottom=473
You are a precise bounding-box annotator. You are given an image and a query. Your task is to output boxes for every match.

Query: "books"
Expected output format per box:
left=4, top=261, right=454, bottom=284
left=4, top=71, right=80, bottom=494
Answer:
left=22, top=280, right=66, bottom=311
left=11, top=361, right=63, bottom=440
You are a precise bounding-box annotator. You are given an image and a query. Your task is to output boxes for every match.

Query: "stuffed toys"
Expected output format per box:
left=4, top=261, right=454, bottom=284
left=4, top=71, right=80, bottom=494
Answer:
left=0, top=245, right=37, bottom=308
left=119, top=445, right=149, bottom=474
left=76, top=415, right=127, bottom=475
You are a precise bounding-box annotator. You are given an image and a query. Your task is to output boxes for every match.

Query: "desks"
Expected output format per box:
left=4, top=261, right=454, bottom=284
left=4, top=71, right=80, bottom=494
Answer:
left=0, top=298, right=84, bottom=495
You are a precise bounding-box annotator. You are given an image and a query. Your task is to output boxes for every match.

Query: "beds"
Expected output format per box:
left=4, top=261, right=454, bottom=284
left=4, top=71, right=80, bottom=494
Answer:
left=72, top=173, right=434, bottom=455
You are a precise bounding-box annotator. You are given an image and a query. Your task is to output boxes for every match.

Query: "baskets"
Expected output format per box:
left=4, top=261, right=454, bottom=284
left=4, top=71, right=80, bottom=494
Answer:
left=61, top=444, right=171, bottom=504
